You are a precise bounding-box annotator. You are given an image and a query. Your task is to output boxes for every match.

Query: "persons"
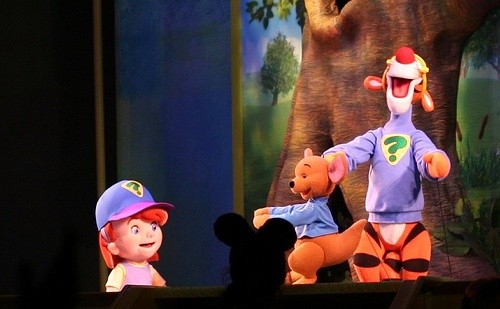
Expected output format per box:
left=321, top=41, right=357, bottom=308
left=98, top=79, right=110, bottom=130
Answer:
left=0, top=213, right=500, bottom=309
